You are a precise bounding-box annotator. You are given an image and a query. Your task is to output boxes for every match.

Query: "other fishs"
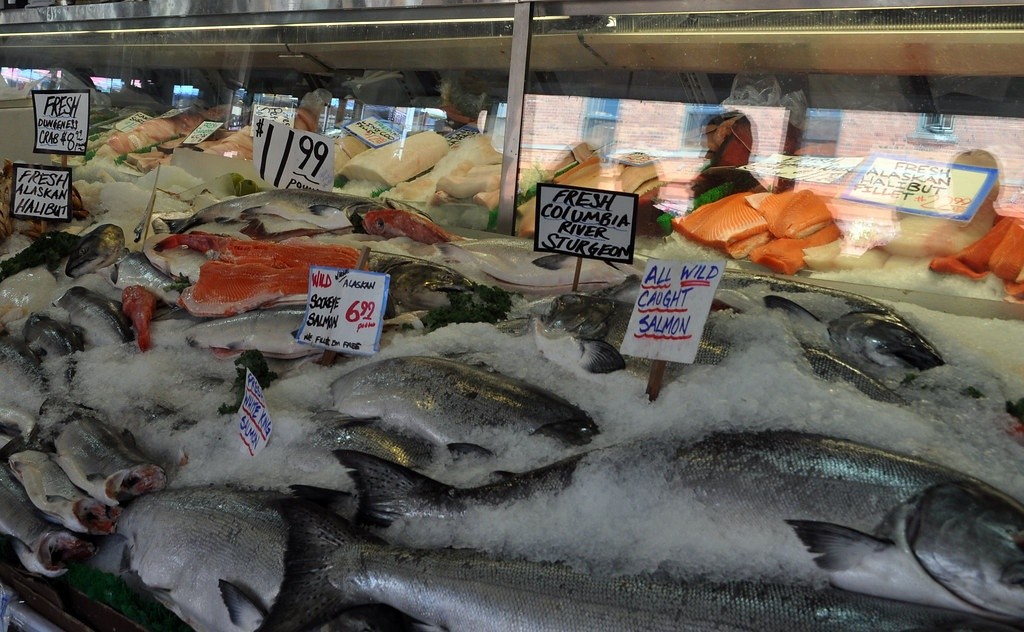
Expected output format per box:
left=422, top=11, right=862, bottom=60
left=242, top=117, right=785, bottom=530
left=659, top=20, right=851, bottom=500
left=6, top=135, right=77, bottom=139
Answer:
left=0, top=188, right=1024, bottom=632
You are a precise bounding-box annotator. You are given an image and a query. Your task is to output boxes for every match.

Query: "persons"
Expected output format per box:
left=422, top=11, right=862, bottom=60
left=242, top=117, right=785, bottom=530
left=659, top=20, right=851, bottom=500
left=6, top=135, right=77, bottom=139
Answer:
left=690, top=110, right=769, bottom=205
left=924, top=148, right=1023, bottom=257
left=865, top=182, right=942, bottom=255
